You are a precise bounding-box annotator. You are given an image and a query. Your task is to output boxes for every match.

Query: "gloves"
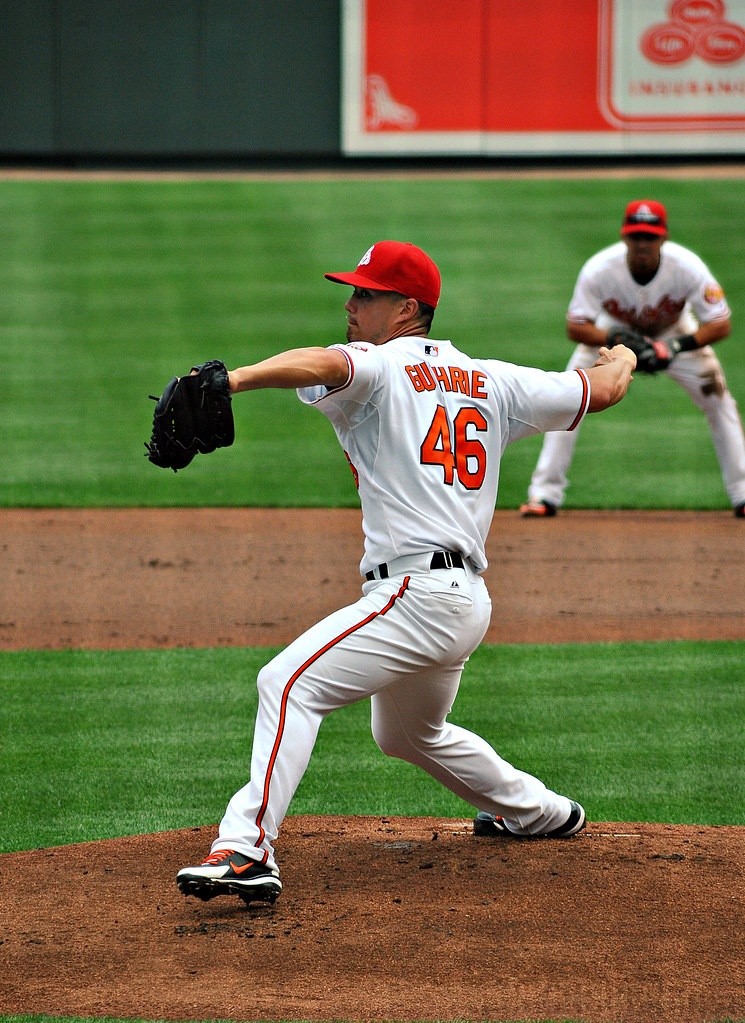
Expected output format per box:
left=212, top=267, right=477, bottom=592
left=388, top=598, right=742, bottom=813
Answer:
left=606, top=325, right=698, bottom=376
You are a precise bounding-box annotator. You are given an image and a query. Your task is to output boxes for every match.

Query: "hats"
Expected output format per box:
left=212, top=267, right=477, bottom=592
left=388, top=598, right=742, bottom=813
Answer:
left=323, top=241, right=441, bottom=308
left=620, top=200, right=669, bottom=235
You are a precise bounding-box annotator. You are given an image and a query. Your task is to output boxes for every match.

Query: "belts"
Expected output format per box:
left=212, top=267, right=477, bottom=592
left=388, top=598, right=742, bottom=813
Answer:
left=366, top=548, right=462, bottom=581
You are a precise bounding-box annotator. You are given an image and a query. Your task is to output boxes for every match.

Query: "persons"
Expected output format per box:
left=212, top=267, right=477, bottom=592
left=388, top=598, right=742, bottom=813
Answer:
left=518, top=198, right=745, bottom=522
left=144, top=239, right=636, bottom=906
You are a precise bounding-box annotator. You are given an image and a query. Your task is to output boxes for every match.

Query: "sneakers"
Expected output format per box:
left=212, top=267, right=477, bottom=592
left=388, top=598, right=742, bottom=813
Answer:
left=176, top=851, right=283, bottom=907
left=474, top=800, right=585, bottom=838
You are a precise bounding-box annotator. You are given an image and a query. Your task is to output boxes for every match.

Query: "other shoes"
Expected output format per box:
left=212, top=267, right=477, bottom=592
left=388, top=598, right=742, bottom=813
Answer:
left=519, top=499, right=557, bottom=516
left=733, top=502, right=745, bottom=520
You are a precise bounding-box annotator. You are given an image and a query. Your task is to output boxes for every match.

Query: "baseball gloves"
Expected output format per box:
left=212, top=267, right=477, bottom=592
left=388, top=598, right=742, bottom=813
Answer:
left=145, top=359, right=235, bottom=469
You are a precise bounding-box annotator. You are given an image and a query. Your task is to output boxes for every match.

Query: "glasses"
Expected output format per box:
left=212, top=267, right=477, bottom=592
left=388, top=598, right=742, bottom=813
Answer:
left=624, top=212, right=664, bottom=226
